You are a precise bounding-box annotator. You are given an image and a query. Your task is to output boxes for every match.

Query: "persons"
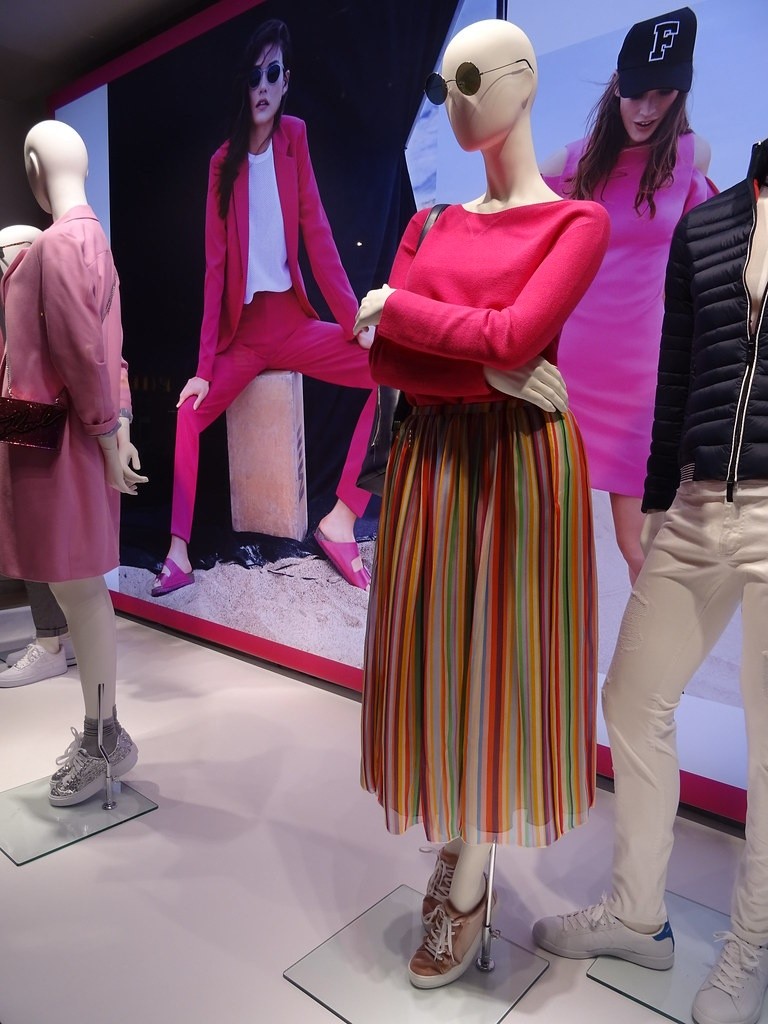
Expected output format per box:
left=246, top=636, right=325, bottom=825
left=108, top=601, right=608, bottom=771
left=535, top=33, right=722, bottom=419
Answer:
left=532, top=145, right=768, bottom=1024
left=151, top=17, right=375, bottom=596
left=545, top=7, right=712, bottom=586
left=0, top=120, right=150, bottom=806
left=353, top=20, right=598, bottom=990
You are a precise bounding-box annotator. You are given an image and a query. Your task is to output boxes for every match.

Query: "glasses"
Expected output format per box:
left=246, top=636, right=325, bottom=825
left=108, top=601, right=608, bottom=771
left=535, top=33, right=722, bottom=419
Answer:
left=245, top=64, right=285, bottom=90
left=0, top=242, right=34, bottom=261
left=421, top=57, right=537, bottom=104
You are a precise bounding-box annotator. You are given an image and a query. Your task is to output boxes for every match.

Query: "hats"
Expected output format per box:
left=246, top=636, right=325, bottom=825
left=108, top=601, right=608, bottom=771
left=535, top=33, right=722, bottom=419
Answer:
left=618, top=2, right=697, bottom=102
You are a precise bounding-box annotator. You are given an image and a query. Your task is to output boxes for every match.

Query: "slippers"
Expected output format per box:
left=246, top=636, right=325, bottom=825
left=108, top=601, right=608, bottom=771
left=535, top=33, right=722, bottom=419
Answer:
left=314, top=527, right=372, bottom=591
left=151, top=558, right=194, bottom=596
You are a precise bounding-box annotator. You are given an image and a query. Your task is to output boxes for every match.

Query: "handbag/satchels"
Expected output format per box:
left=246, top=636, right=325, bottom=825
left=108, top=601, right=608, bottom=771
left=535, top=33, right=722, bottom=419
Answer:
left=355, top=385, right=410, bottom=495
left=1, top=396, right=69, bottom=452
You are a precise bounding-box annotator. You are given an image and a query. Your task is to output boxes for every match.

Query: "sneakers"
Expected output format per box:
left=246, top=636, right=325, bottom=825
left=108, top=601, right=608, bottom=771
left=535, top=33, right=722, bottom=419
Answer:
left=48, top=727, right=138, bottom=807
left=408, top=845, right=499, bottom=989
left=690, top=932, right=768, bottom=1023
left=0, top=637, right=77, bottom=689
left=532, top=889, right=676, bottom=973
